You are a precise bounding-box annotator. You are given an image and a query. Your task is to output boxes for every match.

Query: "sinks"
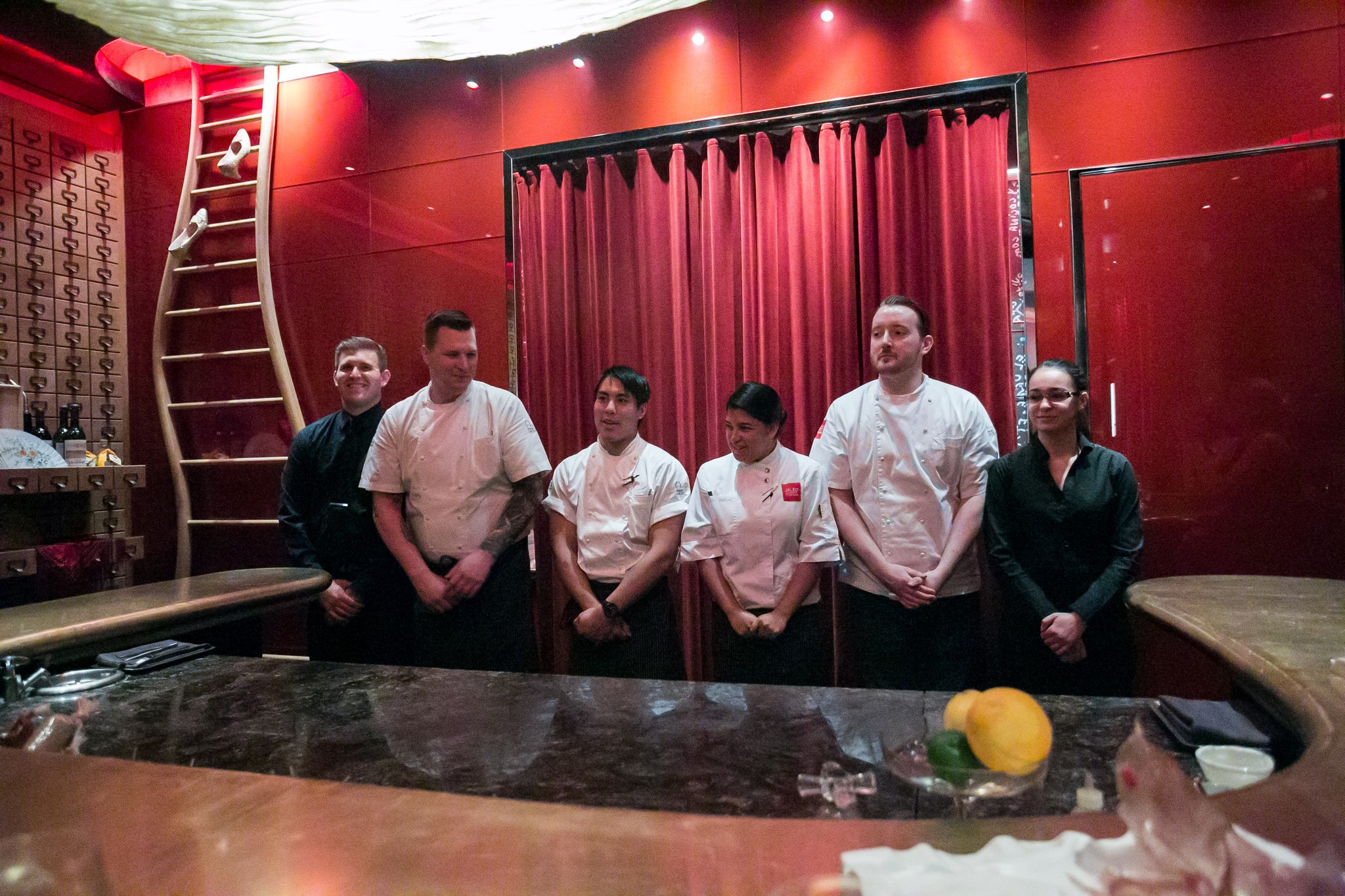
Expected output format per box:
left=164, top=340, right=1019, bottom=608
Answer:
left=26, top=668, right=125, bottom=695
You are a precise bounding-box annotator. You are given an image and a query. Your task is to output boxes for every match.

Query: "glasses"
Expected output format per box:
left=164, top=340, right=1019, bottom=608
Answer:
left=1024, top=391, right=1079, bottom=405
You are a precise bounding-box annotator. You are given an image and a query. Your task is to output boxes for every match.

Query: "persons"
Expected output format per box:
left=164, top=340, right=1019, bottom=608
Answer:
left=539, top=365, right=690, bottom=679
left=278, top=336, right=417, bottom=666
left=676, top=381, right=846, bottom=687
left=289, top=661, right=1145, bottom=822
left=809, top=296, right=1000, bottom=691
left=357, top=309, right=552, bottom=673
left=980, top=357, right=1145, bottom=695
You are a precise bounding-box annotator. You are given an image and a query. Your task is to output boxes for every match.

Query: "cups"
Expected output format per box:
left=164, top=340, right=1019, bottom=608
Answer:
left=1195, top=745, right=1275, bottom=790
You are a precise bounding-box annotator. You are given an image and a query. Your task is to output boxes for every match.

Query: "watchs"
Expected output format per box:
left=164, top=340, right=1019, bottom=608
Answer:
left=600, top=600, right=621, bottom=618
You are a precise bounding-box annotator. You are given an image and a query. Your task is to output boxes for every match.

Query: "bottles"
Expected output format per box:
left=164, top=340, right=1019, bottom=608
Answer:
left=52, top=407, right=69, bottom=459
left=32, top=408, right=52, bottom=446
left=64, top=404, right=86, bottom=467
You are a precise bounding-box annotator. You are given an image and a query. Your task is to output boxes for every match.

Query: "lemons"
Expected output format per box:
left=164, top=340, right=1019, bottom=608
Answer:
left=927, top=729, right=978, bottom=783
left=944, top=690, right=983, bottom=732
left=966, top=687, right=1052, bottom=774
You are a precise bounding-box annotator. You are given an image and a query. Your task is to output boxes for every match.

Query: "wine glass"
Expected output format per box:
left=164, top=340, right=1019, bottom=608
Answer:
left=879, top=714, right=1049, bottom=817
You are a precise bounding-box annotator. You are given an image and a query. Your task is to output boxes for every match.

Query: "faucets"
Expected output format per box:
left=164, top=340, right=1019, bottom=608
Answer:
left=0, top=656, right=50, bottom=703
